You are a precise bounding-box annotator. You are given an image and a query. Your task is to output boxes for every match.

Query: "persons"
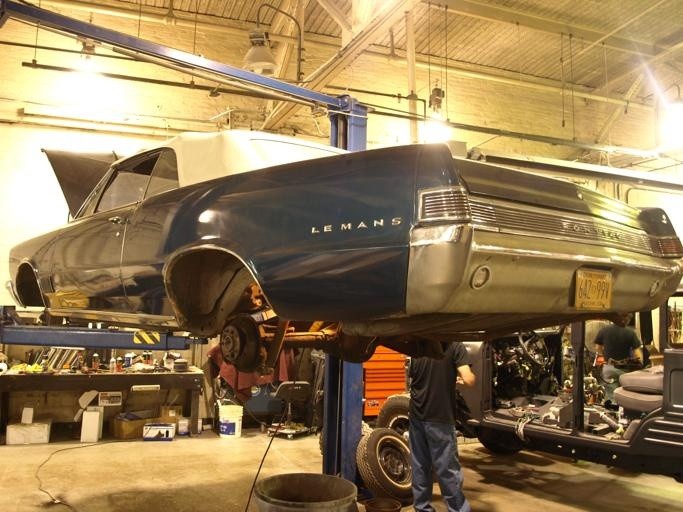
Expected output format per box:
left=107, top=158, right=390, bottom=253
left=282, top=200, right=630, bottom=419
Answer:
left=407, top=340, right=477, bottom=512
left=594, top=312, right=646, bottom=402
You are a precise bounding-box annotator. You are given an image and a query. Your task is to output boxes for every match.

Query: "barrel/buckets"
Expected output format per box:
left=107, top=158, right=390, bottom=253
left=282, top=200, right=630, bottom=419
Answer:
left=218, top=403, right=244, bottom=439
left=251, top=471, right=358, bottom=512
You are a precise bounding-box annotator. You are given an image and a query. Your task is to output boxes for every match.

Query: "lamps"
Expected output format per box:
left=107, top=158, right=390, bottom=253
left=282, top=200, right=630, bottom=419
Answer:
left=241, top=3, right=307, bottom=85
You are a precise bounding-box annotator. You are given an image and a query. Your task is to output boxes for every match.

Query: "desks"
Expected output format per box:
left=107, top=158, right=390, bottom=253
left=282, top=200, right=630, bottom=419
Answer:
left=0, top=371, right=204, bottom=452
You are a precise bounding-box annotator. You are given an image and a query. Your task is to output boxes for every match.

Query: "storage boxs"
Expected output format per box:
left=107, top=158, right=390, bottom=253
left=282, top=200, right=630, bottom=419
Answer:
left=6, top=390, right=192, bottom=444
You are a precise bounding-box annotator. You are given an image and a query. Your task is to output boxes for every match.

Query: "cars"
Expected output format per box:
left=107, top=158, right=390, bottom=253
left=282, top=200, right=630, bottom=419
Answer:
left=4, top=127, right=683, bottom=373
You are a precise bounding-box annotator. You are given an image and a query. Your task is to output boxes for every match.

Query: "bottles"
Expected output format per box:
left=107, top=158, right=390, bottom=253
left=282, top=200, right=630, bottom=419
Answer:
left=142, top=351, right=152, bottom=364
left=91, top=351, right=100, bottom=369
left=125, top=353, right=132, bottom=368
left=109, top=355, right=122, bottom=375
left=41, top=355, right=49, bottom=371
